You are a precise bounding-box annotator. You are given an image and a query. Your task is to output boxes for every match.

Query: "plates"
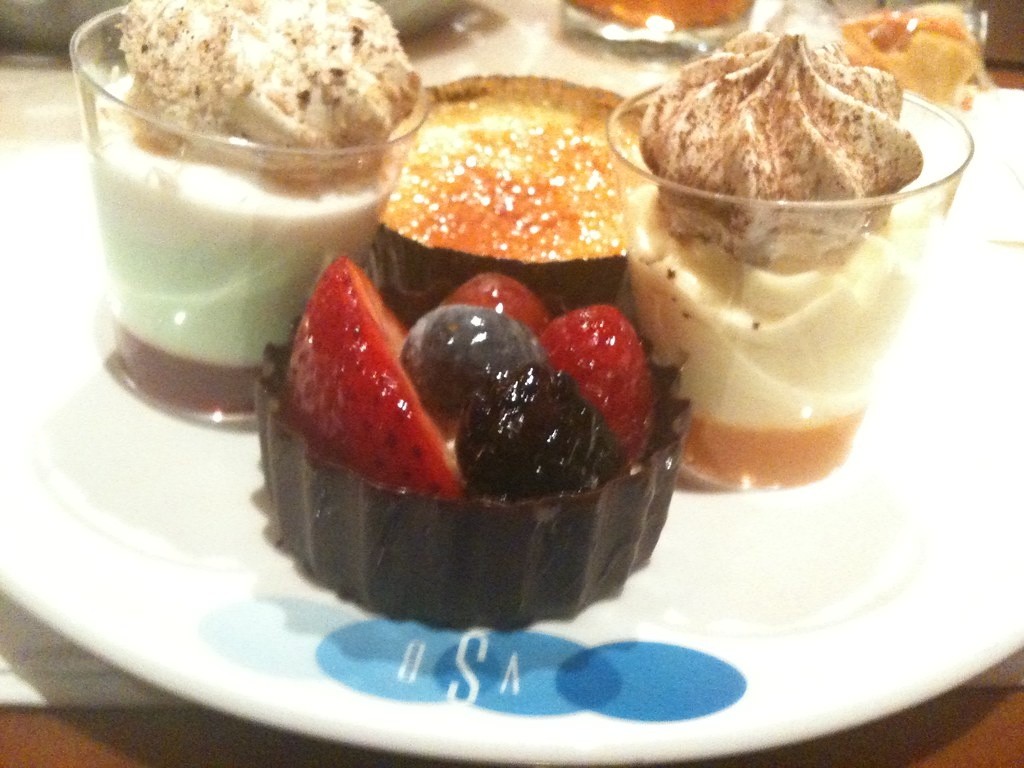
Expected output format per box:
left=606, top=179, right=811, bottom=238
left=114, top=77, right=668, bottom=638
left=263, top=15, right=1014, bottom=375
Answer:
left=1, top=2, right=1023, bottom=765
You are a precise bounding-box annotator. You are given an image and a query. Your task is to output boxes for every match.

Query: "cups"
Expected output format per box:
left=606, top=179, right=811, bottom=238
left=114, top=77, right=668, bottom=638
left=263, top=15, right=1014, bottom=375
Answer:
left=67, top=9, right=429, bottom=430
left=607, top=80, right=975, bottom=492
left=559, top=1, right=757, bottom=56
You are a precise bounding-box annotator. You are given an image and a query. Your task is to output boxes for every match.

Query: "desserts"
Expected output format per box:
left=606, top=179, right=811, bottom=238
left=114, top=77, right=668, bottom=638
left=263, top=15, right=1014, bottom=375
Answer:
left=627, top=29, right=914, bottom=488
left=97, top=0, right=421, bottom=413
left=253, top=255, right=693, bottom=625
